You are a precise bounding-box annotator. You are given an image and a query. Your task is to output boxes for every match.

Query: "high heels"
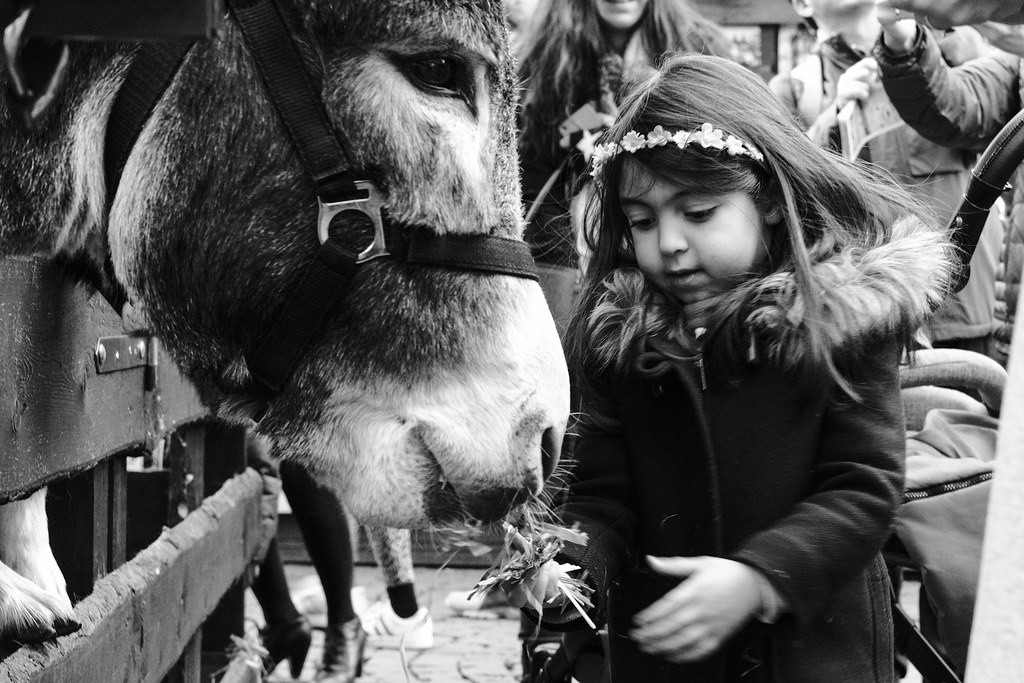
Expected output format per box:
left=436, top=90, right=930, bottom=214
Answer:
left=316, top=616, right=366, bottom=683
left=258, top=614, right=312, bottom=679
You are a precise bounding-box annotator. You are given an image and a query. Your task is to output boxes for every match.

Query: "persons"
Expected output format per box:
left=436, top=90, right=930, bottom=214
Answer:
left=253, top=0, right=1024, bottom=683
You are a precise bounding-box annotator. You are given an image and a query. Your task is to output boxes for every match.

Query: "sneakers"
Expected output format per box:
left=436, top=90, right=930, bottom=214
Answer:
left=360, top=598, right=434, bottom=648
left=444, top=588, right=522, bottom=620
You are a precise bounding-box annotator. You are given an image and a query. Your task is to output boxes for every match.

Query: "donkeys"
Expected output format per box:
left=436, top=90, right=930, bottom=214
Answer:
left=0, top=0, right=571, bottom=643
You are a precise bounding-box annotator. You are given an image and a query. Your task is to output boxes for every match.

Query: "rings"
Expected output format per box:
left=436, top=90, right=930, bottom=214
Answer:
left=895, top=8, right=901, bottom=20
